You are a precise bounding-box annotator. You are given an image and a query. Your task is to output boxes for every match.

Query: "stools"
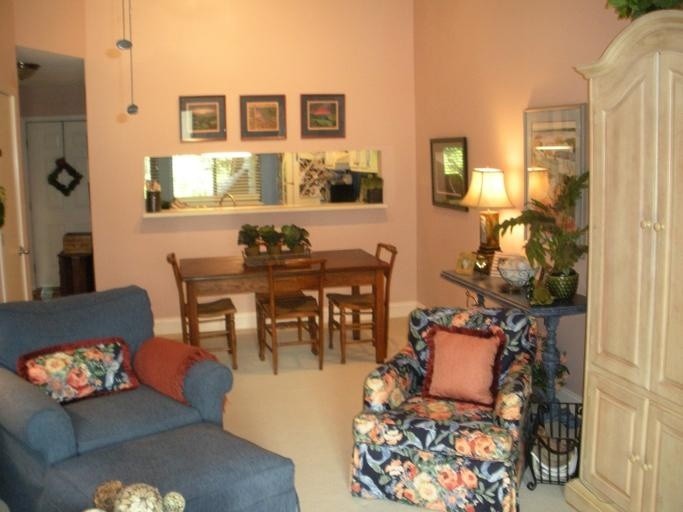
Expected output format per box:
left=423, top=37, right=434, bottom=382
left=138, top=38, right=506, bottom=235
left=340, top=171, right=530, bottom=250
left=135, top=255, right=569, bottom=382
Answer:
left=36, top=420, right=301, bottom=512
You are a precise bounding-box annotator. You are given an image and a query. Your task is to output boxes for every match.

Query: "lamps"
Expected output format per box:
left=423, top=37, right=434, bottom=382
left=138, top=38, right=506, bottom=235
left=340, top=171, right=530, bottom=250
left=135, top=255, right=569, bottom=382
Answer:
left=18, top=60, right=41, bottom=80
left=457, top=168, right=515, bottom=275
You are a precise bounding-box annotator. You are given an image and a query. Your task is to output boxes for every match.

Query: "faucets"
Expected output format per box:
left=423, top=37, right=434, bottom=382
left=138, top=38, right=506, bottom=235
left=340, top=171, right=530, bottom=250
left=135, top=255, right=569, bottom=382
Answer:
left=219, top=194, right=237, bottom=206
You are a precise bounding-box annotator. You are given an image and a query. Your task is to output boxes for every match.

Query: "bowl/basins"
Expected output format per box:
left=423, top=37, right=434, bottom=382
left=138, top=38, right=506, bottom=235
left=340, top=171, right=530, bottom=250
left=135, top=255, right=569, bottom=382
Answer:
left=496, top=264, right=539, bottom=291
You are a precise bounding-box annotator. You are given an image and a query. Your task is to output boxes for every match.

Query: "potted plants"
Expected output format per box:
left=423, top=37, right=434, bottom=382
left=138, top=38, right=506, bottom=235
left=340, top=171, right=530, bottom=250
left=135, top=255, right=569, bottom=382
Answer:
left=492, top=171, right=589, bottom=304
left=359, top=176, right=382, bottom=203
left=237, top=224, right=312, bottom=267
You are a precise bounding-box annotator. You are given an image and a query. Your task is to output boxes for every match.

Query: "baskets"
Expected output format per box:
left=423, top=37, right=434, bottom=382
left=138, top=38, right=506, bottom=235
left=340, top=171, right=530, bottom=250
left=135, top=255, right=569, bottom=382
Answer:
left=527, top=400, right=583, bottom=491
left=498, top=267, right=539, bottom=287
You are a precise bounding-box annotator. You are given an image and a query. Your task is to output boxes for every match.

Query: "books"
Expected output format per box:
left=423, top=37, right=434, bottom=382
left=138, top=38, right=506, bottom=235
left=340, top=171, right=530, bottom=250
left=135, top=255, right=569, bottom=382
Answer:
left=530, top=445, right=578, bottom=483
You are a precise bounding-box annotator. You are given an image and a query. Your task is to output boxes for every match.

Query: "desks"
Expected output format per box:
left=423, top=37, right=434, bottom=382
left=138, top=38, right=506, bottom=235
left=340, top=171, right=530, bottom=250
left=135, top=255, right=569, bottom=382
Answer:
left=441, top=270, right=587, bottom=428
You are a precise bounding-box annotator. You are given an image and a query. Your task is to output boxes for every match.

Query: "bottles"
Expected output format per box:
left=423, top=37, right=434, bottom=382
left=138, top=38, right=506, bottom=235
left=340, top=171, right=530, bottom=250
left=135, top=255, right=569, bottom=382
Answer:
left=146, top=175, right=160, bottom=212
left=365, top=172, right=383, bottom=204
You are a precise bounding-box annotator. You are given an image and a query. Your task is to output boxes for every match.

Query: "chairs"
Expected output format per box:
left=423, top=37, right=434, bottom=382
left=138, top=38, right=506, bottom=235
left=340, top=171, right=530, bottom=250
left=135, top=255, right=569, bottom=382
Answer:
left=166, top=242, right=398, bottom=375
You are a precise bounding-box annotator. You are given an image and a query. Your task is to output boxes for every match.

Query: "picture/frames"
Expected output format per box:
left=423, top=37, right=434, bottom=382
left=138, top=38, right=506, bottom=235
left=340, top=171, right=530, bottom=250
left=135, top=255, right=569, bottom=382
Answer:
left=524, top=104, right=589, bottom=260
left=240, top=95, right=286, bottom=141
left=179, top=96, right=226, bottom=143
left=301, top=94, right=345, bottom=138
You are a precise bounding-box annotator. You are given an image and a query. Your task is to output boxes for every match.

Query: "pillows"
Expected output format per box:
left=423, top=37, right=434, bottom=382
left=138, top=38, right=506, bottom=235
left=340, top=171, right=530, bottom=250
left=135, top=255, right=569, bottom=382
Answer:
left=421, top=325, right=507, bottom=409
left=16, top=336, right=139, bottom=406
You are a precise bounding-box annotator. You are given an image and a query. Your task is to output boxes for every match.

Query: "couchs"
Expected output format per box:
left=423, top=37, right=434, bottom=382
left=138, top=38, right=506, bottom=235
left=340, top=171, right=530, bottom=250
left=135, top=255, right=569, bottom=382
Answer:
left=1, top=284, right=234, bottom=511
left=349, top=308, right=538, bottom=511
